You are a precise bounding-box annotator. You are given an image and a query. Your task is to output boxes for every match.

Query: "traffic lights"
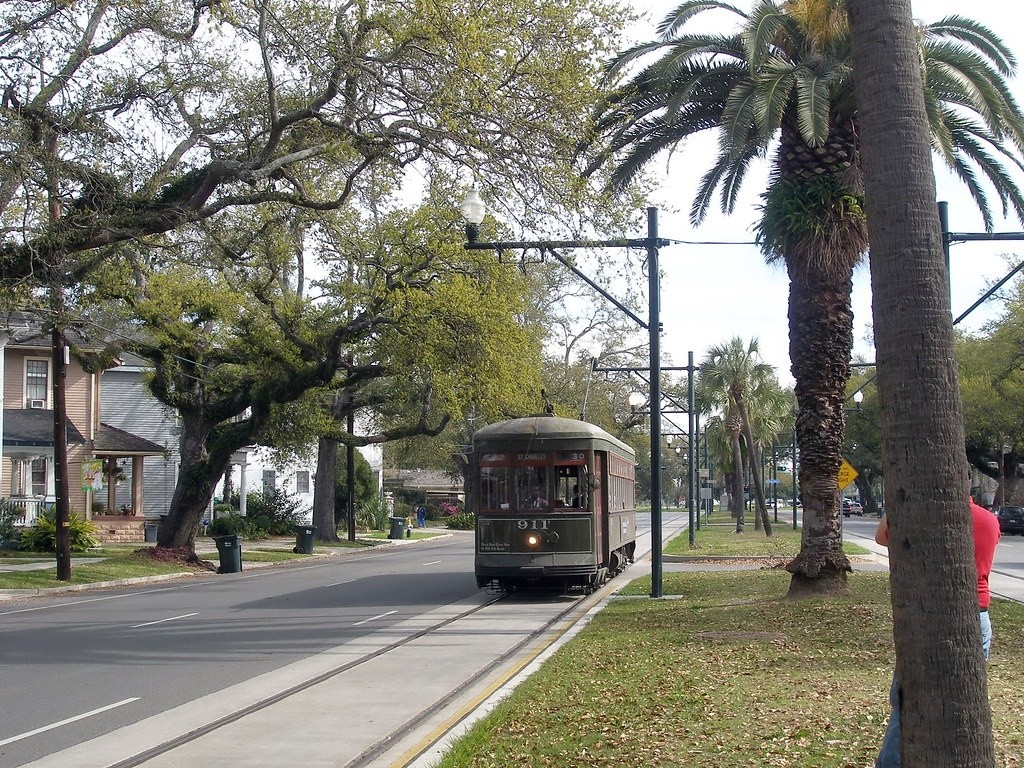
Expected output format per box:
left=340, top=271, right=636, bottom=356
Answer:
left=778, top=467, right=787, bottom=472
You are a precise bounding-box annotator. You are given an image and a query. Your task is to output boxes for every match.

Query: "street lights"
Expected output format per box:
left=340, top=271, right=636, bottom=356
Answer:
left=626, top=391, right=703, bottom=532
left=584, top=334, right=696, bottom=547
left=462, top=180, right=666, bottom=598
left=666, top=436, right=714, bottom=530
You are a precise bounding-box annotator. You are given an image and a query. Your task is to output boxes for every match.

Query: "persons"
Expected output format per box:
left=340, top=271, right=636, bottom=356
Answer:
left=874, top=461, right=1001, bottom=768
left=417, top=505, right=426, bottom=529
left=521, top=485, right=548, bottom=509
left=572, top=485, right=589, bottom=508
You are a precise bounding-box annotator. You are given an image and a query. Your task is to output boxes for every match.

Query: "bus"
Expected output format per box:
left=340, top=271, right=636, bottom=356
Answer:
left=465, top=388, right=637, bottom=598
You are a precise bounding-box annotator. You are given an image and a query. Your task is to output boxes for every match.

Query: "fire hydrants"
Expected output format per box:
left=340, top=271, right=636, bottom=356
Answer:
left=406, top=517, right=415, bottom=530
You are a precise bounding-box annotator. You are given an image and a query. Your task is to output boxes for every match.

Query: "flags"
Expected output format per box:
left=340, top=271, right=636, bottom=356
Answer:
left=80, top=459, right=102, bottom=492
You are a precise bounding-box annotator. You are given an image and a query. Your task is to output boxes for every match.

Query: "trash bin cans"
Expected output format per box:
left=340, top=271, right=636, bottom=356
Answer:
left=145, top=524, right=159, bottom=542
left=212, top=535, right=244, bottom=574
left=388, top=517, right=406, bottom=539
left=291, top=524, right=317, bottom=555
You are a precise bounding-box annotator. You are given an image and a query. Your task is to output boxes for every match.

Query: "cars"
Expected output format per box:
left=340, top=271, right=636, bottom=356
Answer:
left=851, top=503, right=864, bottom=517
left=842, top=501, right=851, bottom=518
left=766, top=501, right=784, bottom=510
left=997, top=506, right=1024, bottom=536
